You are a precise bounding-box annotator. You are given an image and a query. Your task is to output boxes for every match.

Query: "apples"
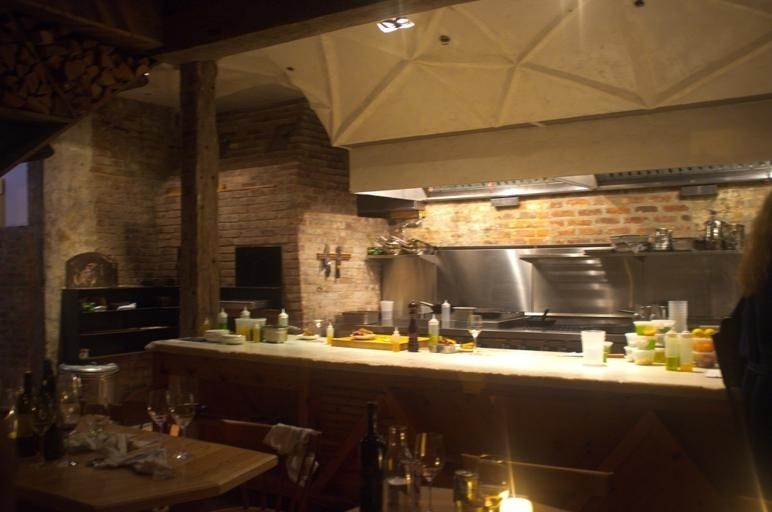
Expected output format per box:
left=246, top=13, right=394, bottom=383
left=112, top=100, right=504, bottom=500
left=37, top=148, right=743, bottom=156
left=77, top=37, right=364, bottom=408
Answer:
left=692, top=328, right=703, bottom=336
left=704, top=329, right=714, bottom=336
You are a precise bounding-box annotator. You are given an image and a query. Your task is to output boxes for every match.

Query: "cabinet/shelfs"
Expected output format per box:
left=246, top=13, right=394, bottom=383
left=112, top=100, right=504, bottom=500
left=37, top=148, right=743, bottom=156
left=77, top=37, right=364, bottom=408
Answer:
left=59, top=283, right=178, bottom=361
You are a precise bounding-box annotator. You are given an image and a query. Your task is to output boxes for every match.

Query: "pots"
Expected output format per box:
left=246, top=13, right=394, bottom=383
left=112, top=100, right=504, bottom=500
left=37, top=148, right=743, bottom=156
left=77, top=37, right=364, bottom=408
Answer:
left=522, top=308, right=556, bottom=327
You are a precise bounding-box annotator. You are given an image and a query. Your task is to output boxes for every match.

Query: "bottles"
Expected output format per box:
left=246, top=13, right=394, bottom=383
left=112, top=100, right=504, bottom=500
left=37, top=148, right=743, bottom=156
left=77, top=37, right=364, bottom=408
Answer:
left=251, top=322, right=261, bottom=342
left=359, top=401, right=386, bottom=510
left=201, top=315, right=212, bottom=339
left=278, top=308, right=288, bottom=342
left=440, top=299, right=451, bottom=322
left=407, top=300, right=419, bottom=353
left=325, top=321, right=334, bottom=344
left=390, top=327, right=401, bottom=352
left=13, top=356, right=55, bottom=463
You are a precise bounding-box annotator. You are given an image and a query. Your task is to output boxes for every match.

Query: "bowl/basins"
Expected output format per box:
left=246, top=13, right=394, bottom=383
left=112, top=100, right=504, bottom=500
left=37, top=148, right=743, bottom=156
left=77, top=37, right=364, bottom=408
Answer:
left=620, top=319, right=673, bottom=365
left=692, top=337, right=716, bottom=369
left=602, top=342, right=614, bottom=363
left=235, top=317, right=265, bottom=342
left=263, top=324, right=288, bottom=344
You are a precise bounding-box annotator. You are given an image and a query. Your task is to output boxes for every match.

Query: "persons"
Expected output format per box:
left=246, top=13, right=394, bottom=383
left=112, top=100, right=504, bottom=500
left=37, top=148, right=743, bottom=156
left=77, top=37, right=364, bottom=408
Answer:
left=712, top=189, right=772, bottom=512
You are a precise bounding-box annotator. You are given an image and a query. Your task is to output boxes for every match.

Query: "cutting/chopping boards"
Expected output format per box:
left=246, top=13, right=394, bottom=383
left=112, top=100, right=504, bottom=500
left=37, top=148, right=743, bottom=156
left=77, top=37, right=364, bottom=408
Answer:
left=330, top=332, right=435, bottom=352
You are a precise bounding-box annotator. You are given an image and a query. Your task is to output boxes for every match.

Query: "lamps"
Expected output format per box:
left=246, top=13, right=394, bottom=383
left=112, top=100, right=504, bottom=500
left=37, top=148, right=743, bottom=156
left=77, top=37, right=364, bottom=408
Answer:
left=375, top=17, right=418, bottom=38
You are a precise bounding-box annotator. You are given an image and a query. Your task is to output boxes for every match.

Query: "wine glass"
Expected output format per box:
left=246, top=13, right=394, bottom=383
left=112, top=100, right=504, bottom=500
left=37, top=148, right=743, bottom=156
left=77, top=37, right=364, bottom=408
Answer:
left=144, top=383, right=198, bottom=459
left=390, top=425, right=509, bottom=511
left=464, top=313, right=484, bottom=355
left=39, top=375, right=115, bottom=467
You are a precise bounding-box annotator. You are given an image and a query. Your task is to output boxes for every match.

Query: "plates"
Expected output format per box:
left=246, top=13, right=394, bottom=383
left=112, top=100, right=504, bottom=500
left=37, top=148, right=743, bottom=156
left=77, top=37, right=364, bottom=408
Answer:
left=204, top=329, right=247, bottom=345
left=298, top=334, right=319, bottom=340
left=452, top=343, right=478, bottom=352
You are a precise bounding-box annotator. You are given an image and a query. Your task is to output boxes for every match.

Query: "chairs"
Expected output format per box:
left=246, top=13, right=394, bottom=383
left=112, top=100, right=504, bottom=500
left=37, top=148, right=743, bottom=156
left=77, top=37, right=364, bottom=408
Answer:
left=457, top=450, right=614, bottom=511
left=210, top=417, right=324, bottom=511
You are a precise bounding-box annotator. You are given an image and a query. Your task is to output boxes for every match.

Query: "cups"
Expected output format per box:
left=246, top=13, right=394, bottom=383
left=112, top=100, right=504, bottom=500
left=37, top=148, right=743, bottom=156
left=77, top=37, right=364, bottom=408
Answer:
left=580, top=329, right=604, bottom=366
left=662, top=333, right=693, bottom=373
left=667, top=300, right=688, bottom=332
left=379, top=299, right=395, bottom=319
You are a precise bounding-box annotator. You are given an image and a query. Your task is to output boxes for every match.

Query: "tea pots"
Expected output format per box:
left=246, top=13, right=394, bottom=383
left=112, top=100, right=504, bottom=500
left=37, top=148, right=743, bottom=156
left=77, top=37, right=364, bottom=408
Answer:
left=698, top=218, right=746, bottom=251
left=651, top=228, right=676, bottom=250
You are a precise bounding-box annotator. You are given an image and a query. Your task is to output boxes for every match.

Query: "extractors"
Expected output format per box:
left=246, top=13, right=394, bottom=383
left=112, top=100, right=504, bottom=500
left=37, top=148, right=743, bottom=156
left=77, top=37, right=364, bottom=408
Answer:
left=345, top=98, right=769, bottom=208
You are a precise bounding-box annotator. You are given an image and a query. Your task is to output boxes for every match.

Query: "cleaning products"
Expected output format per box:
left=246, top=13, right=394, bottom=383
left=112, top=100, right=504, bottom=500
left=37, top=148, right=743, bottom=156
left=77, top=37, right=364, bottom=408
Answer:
left=253, top=321, right=260, bottom=342
left=327, top=319, right=334, bottom=344
left=440, top=301, right=450, bottom=329
left=393, top=327, right=400, bottom=352
left=278, top=308, right=289, bottom=327
